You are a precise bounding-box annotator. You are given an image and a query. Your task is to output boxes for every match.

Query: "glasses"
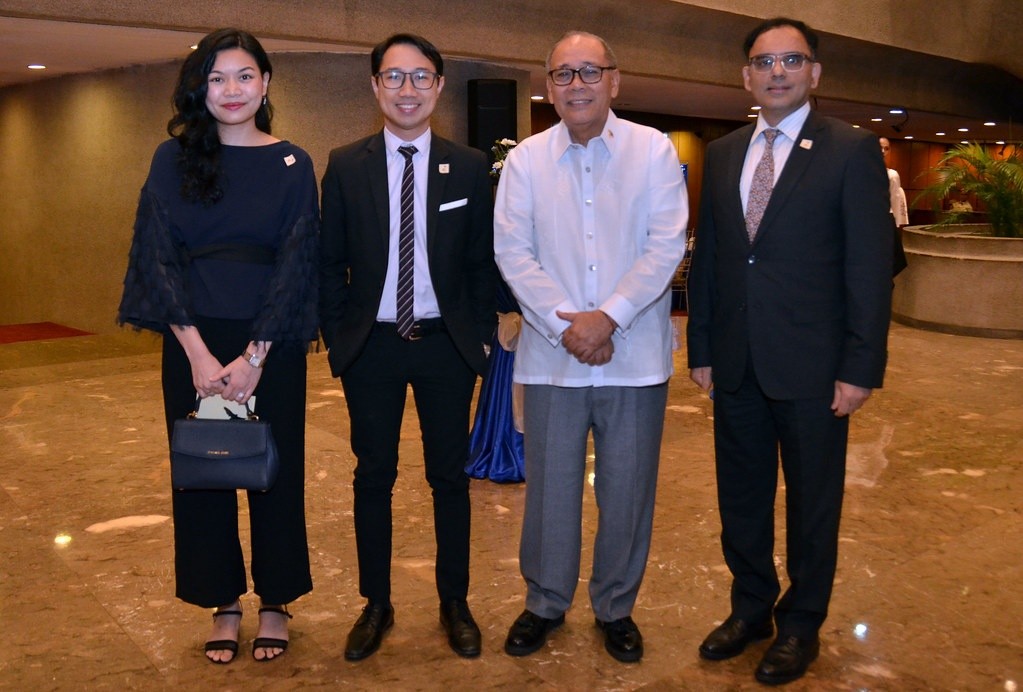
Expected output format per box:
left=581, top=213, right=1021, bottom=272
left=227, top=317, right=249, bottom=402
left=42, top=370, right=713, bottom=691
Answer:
left=747, top=51, right=814, bottom=72
left=547, top=65, right=615, bottom=86
left=375, top=69, right=442, bottom=89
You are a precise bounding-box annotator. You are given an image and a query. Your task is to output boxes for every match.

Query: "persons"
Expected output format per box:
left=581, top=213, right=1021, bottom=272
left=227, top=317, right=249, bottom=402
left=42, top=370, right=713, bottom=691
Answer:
left=879, top=136, right=908, bottom=277
left=949, top=191, right=972, bottom=210
left=118, top=28, right=319, bottom=664
left=688, top=17, right=909, bottom=684
left=320, top=30, right=518, bottom=657
left=494, top=30, right=689, bottom=662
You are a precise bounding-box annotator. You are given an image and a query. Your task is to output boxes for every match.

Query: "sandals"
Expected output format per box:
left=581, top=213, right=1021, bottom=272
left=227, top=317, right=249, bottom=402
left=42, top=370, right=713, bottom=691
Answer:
left=204, top=598, right=242, bottom=665
left=252, top=604, right=292, bottom=662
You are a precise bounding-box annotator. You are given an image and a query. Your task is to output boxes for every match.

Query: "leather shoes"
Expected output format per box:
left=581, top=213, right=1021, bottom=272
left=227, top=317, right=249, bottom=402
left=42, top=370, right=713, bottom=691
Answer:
left=439, top=606, right=481, bottom=657
left=755, top=631, right=819, bottom=686
left=505, top=609, right=565, bottom=656
left=698, top=612, right=774, bottom=661
left=594, top=616, right=643, bottom=663
left=344, top=603, right=395, bottom=660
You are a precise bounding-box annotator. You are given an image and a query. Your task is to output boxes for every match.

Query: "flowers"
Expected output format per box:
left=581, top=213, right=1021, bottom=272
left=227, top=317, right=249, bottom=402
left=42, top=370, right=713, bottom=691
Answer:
left=489, top=138, right=518, bottom=175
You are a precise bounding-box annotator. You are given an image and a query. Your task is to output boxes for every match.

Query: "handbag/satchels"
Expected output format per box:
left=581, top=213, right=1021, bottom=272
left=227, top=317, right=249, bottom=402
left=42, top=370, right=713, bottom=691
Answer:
left=171, top=381, right=278, bottom=493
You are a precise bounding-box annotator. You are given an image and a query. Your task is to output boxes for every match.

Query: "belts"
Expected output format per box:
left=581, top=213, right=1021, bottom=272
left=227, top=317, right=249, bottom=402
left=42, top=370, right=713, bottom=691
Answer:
left=373, top=316, right=443, bottom=340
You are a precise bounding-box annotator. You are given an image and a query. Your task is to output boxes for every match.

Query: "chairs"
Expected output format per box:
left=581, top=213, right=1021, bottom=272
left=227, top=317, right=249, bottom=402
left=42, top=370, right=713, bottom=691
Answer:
left=672, top=227, right=695, bottom=314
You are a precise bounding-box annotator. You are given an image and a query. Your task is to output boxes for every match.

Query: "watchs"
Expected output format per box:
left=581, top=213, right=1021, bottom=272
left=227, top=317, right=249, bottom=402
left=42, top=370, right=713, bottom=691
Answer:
left=242, top=351, right=265, bottom=368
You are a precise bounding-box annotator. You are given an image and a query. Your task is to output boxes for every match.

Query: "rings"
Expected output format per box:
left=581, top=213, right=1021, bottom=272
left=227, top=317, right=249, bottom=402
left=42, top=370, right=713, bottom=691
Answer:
left=237, top=392, right=244, bottom=400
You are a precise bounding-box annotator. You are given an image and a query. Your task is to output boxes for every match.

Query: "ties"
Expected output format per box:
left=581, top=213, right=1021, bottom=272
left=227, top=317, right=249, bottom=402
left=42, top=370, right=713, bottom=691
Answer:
left=745, top=127, right=783, bottom=244
left=396, top=146, right=418, bottom=339
